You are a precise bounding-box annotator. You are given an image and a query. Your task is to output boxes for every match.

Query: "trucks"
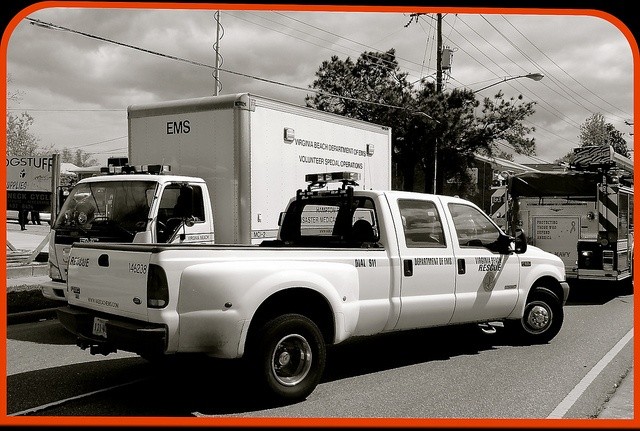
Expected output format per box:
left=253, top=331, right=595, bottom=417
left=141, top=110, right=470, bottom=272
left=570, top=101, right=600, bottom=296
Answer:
left=40, top=92, right=392, bottom=301
left=490, top=145, right=633, bottom=282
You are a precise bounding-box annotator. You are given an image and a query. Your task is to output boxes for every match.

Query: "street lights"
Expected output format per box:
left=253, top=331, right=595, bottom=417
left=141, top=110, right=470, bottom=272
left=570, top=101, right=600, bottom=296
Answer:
left=411, top=73, right=544, bottom=192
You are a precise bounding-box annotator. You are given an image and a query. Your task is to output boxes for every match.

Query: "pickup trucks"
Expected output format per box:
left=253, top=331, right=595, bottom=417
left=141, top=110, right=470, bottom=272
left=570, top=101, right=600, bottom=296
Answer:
left=57, top=172, right=569, bottom=401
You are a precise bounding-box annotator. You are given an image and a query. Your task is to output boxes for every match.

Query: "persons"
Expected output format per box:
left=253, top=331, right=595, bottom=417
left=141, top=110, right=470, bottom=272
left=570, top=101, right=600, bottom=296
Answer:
left=18, top=211, right=28, bottom=230
left=31, top=210, right=41, bottom=225
